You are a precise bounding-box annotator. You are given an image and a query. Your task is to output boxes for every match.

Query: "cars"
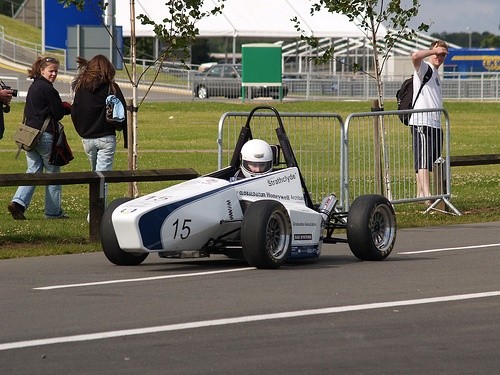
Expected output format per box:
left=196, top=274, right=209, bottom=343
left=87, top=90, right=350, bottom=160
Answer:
left=192, top=65, right=289, bottom=99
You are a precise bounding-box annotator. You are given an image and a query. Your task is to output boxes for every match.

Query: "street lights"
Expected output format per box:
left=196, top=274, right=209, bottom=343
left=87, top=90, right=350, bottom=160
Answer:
left=466, top=26, right=471, bottom=49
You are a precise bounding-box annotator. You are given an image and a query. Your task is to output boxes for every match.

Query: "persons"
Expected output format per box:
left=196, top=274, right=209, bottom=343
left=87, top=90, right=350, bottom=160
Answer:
left=233, top=139, right=273, bottom=181
left=71, top=54, right=126, bottom=222
left=0, top=88, right=14, bottom=139
left=8, top=57, right=71, bottom=220
left=412, top=41, right=449, bottom=206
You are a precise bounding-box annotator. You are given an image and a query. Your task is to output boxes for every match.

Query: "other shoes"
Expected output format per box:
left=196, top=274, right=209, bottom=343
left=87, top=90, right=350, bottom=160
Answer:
left=8, top=202, right=26, bottom=220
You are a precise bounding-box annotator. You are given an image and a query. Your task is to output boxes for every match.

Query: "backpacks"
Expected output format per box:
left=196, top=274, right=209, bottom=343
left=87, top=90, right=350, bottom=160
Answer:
left=395, top=65, right=433, bottom=126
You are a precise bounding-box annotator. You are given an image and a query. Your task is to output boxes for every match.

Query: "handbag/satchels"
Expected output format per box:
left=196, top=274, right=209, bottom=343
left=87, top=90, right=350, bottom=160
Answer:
left=14, top=124, right=41, bottom=151
left=106, top=81, right=125, bottom=123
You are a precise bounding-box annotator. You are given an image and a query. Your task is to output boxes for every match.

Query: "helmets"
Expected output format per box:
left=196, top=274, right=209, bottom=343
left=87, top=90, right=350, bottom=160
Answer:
left=239, top=138, right=273, bottom=178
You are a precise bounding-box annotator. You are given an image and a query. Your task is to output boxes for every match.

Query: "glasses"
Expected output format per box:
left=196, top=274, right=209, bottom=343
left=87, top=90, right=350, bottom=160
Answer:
left=38, top=57, right=59, bottom=71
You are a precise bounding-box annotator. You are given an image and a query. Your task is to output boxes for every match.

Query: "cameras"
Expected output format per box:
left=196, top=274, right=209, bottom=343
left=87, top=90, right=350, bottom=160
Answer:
left=2, top=85, right=17, bottom=97
